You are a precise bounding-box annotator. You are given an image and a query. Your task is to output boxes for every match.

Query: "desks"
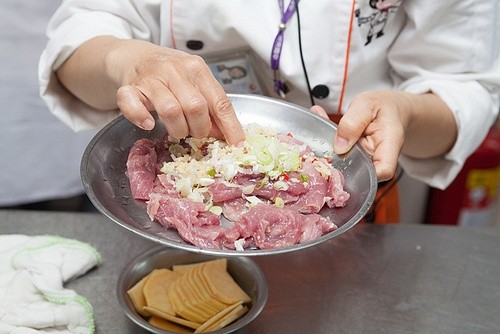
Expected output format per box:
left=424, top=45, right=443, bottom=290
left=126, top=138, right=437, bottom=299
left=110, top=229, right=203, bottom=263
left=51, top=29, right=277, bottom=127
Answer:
left=0, top=206, right=499, bottom=332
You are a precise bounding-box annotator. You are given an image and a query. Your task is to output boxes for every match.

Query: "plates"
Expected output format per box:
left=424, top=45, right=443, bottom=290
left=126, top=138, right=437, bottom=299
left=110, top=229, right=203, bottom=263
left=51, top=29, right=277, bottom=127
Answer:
left=81, top=91, right=379, bottom=256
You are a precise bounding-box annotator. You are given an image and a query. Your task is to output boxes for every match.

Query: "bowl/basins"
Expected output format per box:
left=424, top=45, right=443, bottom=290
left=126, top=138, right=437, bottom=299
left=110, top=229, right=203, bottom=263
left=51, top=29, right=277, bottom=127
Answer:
left=115, top=245, right=268, bottom=334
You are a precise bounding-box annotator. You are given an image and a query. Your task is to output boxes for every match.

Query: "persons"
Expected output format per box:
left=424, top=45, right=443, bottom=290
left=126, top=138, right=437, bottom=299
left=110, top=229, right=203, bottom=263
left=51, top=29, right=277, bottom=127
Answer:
left=36, top=1, right=500, bottom=223
